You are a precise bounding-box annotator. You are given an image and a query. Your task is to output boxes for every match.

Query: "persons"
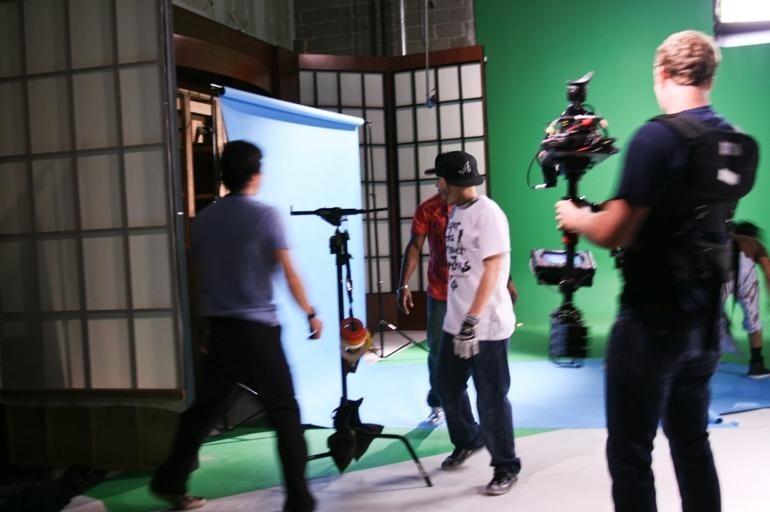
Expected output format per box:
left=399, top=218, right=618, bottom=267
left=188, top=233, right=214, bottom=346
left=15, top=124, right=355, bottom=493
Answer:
left=421, top=146, right=525, bottom=496
left=551, top=26, right=760, bottom=510
left=395, top=149, right=520, bottom=427
left=145, top=137, right=326, bottom=510
left=716, top=232, right=770, bottom=380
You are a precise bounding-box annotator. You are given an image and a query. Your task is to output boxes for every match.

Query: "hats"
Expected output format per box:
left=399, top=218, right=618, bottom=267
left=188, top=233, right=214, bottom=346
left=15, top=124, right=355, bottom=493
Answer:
left=425, top=153, right=443, bottom=176
left=439, top=151, right=486, bottom=185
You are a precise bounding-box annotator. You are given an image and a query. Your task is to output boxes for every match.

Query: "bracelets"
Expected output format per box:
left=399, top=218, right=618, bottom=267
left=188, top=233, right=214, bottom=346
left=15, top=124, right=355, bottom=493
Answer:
left=396, top=284, right=409, bottom=294
left=307, top=312, right=317, bottom=320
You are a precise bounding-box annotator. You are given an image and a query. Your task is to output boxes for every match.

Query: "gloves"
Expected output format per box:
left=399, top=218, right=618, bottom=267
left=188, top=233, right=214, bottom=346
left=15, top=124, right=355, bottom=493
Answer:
left=450, top=314, right=480, bottom=359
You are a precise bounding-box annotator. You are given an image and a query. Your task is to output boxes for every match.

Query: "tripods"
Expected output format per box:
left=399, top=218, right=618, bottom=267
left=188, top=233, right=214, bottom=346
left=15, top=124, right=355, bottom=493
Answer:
left=363, top=119, right=429, bottom=360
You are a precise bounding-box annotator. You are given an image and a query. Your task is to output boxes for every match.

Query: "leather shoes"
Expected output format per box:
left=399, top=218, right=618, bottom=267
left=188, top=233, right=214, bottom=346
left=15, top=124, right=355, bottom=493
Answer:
left=748, top=363, right=770, bottom=380
left=149, top=481, right=206, bottom=509
left=442, top=443, right=485, bottom=471
left=484, top=475, right=519, bottom=495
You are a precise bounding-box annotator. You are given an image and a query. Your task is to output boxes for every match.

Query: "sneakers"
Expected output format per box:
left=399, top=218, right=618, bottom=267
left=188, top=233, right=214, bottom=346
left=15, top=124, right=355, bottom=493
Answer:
left=427, top=406, right=443, bottom=425
